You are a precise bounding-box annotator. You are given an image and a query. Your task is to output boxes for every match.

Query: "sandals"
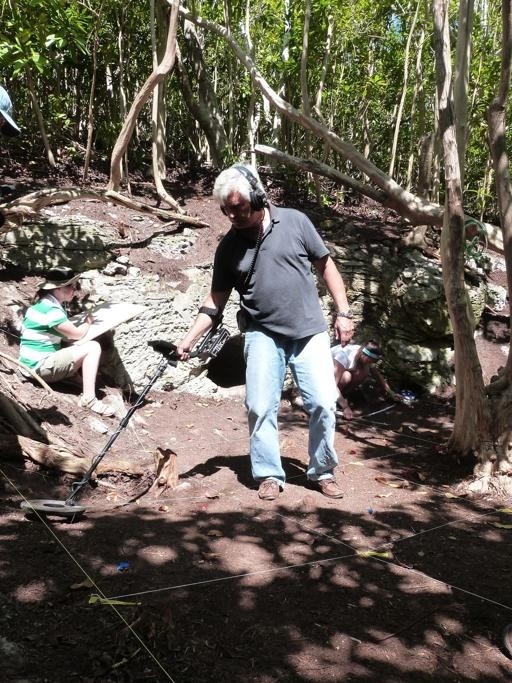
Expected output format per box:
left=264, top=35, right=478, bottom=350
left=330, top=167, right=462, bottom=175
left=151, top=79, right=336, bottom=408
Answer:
left=77, top=393, right=116, bottom=416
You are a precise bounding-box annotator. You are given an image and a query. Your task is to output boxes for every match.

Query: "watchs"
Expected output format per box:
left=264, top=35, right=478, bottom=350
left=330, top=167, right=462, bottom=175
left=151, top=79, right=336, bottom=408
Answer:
left=336, top=310, right=354, bottom=319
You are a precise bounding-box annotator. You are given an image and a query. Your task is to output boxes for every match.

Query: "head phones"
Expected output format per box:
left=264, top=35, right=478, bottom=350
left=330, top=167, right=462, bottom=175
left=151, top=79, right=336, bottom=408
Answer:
left=219, top=165, right=269, bottom=216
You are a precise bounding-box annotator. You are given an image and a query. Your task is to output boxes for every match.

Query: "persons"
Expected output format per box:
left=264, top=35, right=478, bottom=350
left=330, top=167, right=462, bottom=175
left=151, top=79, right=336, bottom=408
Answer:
left=0, top=85, right=21, bottom=137
left=332, top=340, right=401, bottom=421
left=19, top=265, right=115, bottom=416
left=175, top=162, right=355, bottom=501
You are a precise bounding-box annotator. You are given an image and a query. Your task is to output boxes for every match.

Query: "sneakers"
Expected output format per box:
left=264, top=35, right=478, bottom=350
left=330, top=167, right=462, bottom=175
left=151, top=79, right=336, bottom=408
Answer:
left=258, top=479, right=279, bottom=500
left=309, top=478, right=344, bottom=498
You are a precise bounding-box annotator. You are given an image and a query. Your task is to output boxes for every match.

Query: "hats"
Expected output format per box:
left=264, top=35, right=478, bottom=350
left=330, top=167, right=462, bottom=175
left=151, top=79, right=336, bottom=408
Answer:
left=37, top=266, right=81, bottom=290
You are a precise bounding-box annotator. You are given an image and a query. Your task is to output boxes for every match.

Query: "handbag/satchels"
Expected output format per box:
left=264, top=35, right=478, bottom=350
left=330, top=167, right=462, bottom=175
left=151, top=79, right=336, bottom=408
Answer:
left=207, top=333, right=245, bottom=388
